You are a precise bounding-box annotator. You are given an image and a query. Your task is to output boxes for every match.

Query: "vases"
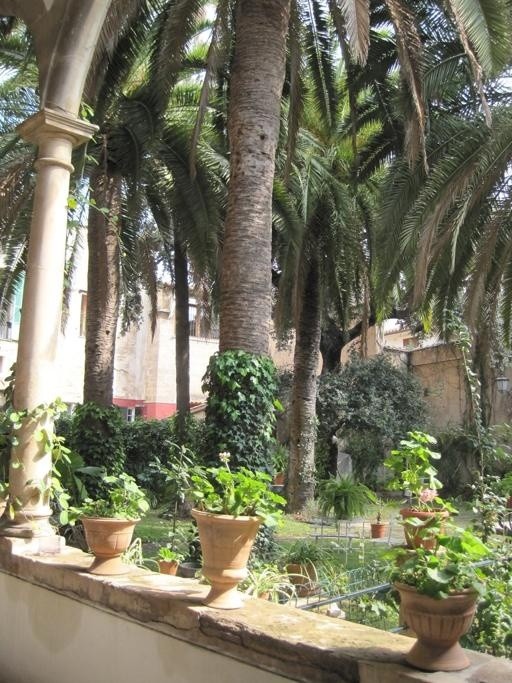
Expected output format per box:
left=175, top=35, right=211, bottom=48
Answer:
left=191, top=507, right=261, bottom=609
left=395, top=579, right=478, bottom=671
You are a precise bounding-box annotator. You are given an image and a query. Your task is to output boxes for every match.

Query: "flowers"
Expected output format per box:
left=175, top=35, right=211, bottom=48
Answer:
left=191, top=450, right=287, bottom=527
left=383, top=488, right=492, bottom=599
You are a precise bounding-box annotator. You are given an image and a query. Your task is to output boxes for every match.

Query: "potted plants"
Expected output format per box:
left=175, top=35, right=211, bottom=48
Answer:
left=273, top=444, right=290, bottom=485
left=500, top=471, right=512, bottom=508
left=69, top=473, right=150, bottom=575
left=159, top=547, right=184, bottom=575
left=246, top=566, right=315, bottom=602
left=316, top=469, right=381, bottom=524
left=284, top=538, right=324, bottom=597
left=382, top=430, right=449, bottom=550
left=370, top=505, right=386, bottom=538
